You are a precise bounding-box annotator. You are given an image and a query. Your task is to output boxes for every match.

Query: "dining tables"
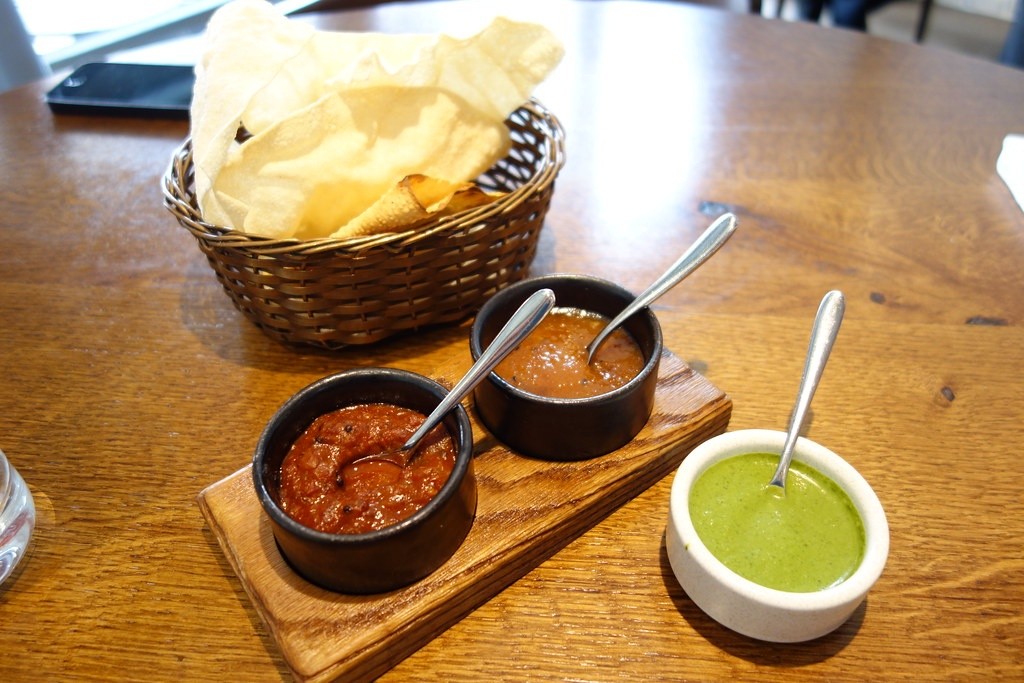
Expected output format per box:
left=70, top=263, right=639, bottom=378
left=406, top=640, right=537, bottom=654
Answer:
left=0, top=0, right=1024, bottom=683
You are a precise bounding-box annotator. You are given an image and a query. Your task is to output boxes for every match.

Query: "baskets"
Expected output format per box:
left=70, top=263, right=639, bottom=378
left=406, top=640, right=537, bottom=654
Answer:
left=161, top=97, right=565, bottom=350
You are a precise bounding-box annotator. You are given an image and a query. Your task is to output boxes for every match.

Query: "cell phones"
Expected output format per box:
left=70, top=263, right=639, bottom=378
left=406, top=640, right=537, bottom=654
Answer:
left=45, top=63, right=195, bottom=119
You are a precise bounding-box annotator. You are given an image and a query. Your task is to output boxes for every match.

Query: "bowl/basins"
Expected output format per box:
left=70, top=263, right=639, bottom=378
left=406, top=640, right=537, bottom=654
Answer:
left=250, top=363, right=479, bottom=597
left=664, top=426, right=891, bottom=644
left=469, top=272, right=666, bottom=461
